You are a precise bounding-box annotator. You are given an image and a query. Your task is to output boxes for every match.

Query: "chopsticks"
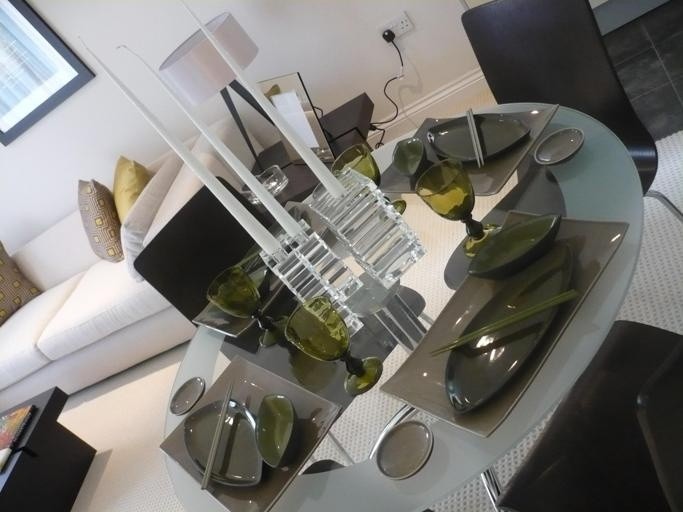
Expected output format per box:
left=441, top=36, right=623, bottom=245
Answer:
left=429, top=288, right=580, bottom=358
left=200, top=381, right=234, bottom=489
left=466, top=107, right=484, bottom=170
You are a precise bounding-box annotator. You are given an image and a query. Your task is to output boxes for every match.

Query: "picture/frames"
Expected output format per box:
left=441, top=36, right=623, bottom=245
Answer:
left=0, top=0, right=96, bottom=147
left=255, top=72, right=336, bottom=164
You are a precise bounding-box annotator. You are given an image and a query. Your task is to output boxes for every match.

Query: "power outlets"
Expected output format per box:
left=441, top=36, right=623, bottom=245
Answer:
left=373, top=10, right=415, bottom=48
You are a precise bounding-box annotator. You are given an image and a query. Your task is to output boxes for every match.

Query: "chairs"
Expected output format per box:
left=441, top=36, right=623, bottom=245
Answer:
left=479, top=321, right=682, bottom=510
left=134, top=178, right=435, bottom=426
left=460, top=0, right=682, bottom=247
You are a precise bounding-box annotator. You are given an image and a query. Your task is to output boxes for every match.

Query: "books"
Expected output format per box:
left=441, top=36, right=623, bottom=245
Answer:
left=0, top=404, right=36, bottom=472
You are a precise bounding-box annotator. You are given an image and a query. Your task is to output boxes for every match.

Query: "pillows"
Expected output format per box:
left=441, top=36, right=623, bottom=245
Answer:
left=113, top=156, right=150, bottom=225
left=120, top=137, right=198, bottom=283
left=1, top=240, right=40, bottom=328
left=78, top=179, right=124, bottom=263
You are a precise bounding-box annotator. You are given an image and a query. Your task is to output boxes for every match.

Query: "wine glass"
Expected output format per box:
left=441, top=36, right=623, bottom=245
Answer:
left=415, top=158, right=502, bottom=258
left=331, top=142, right=407, bottom=219
left=274, top=336, right=338, bottom=394
left=284, top=295, right=383, bottom=397
left=203, top=265, right=291, bottom=348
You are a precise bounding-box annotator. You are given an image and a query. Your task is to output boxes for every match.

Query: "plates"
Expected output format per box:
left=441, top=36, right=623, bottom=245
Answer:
left=444, top=242, right=575, bottom=416
left=375, top=421, right=433, bottom=481
left=168, top=377, right=205, bottom=414
left=205, top=265, right=269, bottom=317
left=184, top=399, right=262, bottom=489
left=534, top=127, right=584, bottom=167
left=468, top=213, right=562, bottom=279
left=427, top=113, right=531, bottom=164
left=391, top=137, right=424, bottom=180
left=255, top=394, right=293, bottom=470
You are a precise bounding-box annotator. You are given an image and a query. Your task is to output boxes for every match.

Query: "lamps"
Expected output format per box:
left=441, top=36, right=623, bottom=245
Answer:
left=158, top=11, right=276, bottom=174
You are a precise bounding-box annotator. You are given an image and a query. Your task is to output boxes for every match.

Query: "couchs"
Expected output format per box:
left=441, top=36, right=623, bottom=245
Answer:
left=0, top=116, right=264, bottom=418
